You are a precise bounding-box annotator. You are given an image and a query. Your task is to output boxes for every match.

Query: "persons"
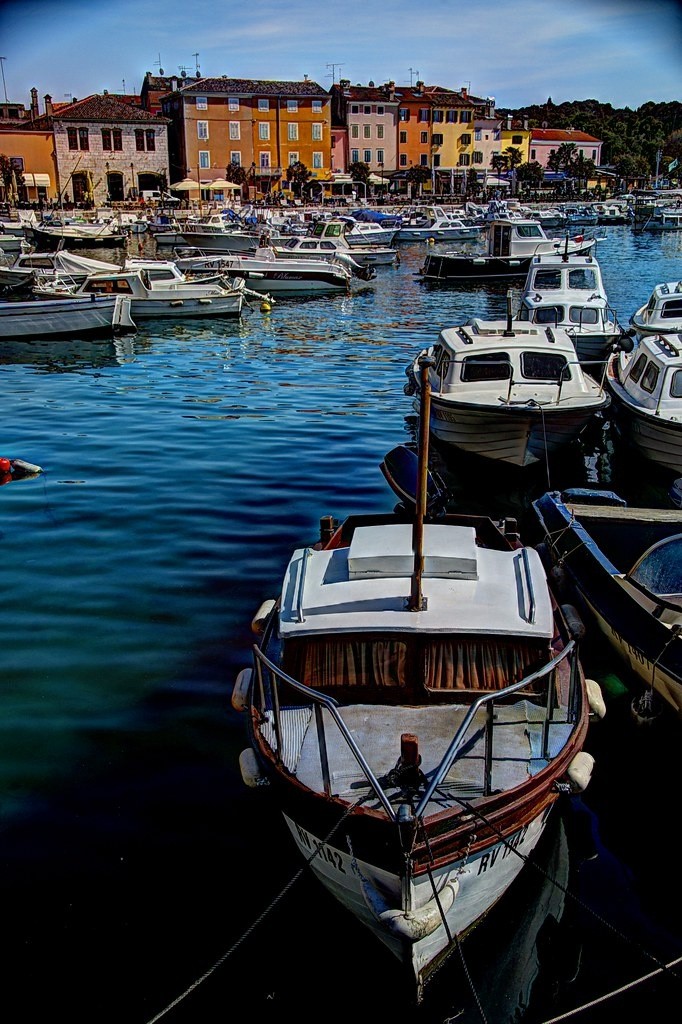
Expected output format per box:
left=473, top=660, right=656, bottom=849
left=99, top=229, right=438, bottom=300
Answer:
left=560, top=185, right=601, bottom=202
left=17, top=196, right=54, bottom=211
left=179, top=198, right=189, bottom=209
left=329, top=192, right=401, bottom=207
left=469, top=187, right=502, bottom=203
left=265, top=189, right=310, bottom=206
left=64, top=192, right=92, bottom=211
left=140, top=197, right=153, bottom=210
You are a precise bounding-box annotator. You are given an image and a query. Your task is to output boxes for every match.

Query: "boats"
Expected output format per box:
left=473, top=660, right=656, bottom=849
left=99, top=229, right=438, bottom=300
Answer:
left=401, top=288, right=614, bottom=495
left=514, top=228, right=622, bottom=368
left=632, top=279, right=682, bottom=337
left=0, top=155, right=682, bottom=342
left=227, top=351, right=607, bottom=1007
left=605, top=332, right=682, bottom=477
left=528, top=486, right=681, bottom=740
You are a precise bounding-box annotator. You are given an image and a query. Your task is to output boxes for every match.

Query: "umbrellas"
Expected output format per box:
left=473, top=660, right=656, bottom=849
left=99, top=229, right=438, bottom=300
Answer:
left=205, top=178, right=242, bottom=190
left=168, top=178, right=208, bottom=190
left=12, top=172, right=19, bottom=201
left=476, top=175, right=510, bottom=186
